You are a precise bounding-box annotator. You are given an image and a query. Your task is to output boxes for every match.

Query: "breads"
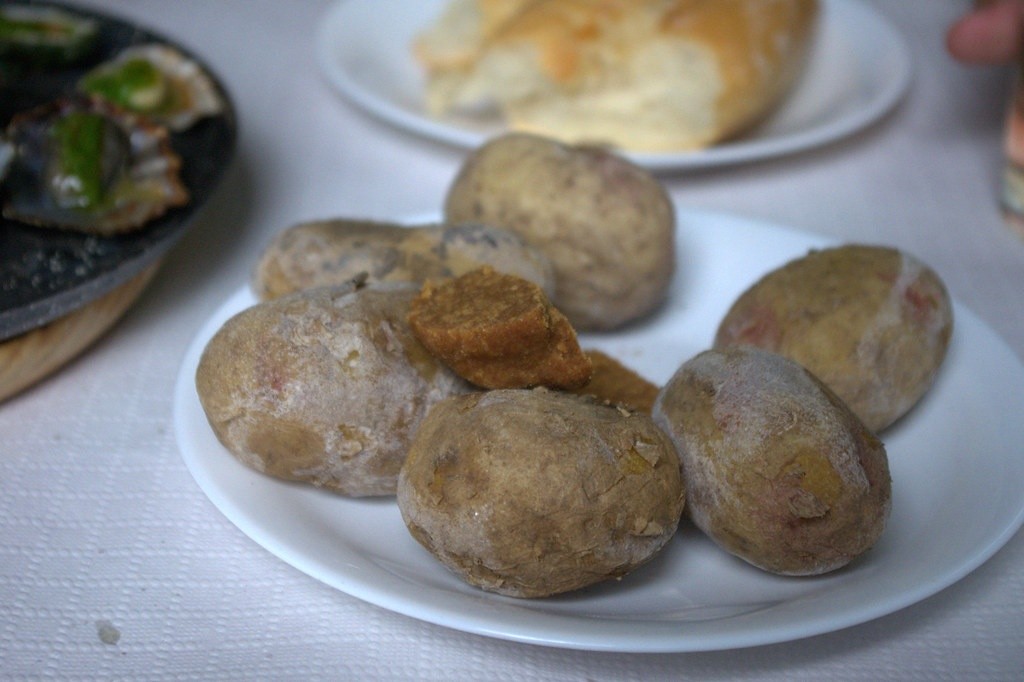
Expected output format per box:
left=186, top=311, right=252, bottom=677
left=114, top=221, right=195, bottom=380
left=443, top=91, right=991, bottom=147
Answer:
left=412, top=0, right=825, bottom=151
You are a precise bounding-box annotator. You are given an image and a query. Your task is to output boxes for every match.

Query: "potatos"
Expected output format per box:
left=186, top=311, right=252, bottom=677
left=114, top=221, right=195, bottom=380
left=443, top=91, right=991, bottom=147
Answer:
left=196, top=135, right=956, bottom=600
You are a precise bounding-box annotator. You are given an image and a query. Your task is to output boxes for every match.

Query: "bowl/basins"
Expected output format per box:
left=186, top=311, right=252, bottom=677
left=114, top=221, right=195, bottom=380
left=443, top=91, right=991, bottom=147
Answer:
left=0, top=0, right=239, bottom=402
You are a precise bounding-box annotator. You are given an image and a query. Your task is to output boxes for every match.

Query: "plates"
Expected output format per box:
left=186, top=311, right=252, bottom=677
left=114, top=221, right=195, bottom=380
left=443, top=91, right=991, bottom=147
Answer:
left=174, top=203, right=1022, bottom=654
left=313, top=0, right=911, bottom=170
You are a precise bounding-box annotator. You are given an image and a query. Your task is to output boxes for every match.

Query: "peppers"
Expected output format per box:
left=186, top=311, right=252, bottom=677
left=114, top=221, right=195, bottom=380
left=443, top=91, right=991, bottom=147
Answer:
left=48, top=58, right=166, bottom=211
